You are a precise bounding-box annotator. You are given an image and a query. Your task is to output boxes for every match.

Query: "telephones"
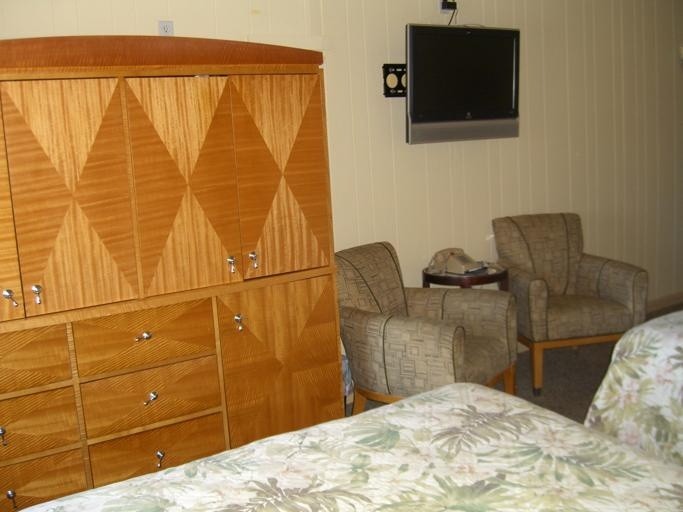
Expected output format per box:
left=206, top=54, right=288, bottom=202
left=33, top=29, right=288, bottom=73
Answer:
left=433, top=248, right=487, bottom=274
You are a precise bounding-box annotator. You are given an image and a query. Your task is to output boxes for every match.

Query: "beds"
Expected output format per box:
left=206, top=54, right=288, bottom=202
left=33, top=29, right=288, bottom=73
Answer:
left=14, top=381, right=682, bottom=512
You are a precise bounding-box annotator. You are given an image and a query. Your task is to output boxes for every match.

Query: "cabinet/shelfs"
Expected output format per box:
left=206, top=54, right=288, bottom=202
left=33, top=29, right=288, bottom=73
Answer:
left=0, top=36, right=347, bottom=512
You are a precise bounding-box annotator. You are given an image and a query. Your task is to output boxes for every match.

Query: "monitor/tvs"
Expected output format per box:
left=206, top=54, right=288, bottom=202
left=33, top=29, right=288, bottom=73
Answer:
left=405, top=22, right=520, bottom=145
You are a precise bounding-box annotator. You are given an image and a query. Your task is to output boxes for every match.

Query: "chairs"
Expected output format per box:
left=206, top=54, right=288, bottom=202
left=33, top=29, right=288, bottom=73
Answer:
left=491, top=211, right=649, bottom=395
left=335, top=241, right=518, bottom=417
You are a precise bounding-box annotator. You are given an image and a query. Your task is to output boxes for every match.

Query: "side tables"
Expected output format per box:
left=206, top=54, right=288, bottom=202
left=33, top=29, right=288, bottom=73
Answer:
left=420, top=260, right=508, bottom=292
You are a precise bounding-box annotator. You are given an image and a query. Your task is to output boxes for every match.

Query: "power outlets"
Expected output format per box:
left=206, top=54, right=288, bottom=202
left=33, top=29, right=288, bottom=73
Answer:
left=157, top=20, right=174, bottom=35
